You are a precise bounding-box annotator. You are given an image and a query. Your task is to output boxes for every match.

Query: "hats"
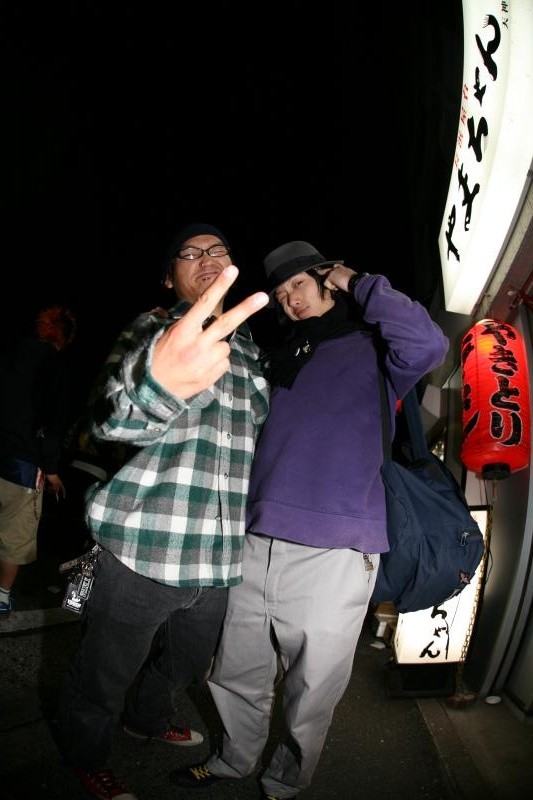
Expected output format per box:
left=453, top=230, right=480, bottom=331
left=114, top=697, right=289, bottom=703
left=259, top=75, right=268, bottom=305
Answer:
left=262, top=240, right=346, bottom=298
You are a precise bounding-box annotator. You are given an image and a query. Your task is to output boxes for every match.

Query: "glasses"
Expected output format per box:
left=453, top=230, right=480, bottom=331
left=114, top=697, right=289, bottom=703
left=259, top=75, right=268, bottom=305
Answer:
left=174, top=243, right=231, bottom=261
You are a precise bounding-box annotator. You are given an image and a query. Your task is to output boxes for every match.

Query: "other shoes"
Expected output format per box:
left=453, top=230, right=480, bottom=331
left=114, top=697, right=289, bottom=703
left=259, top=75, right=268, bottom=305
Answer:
left=0, top=597, right=13, bottom=616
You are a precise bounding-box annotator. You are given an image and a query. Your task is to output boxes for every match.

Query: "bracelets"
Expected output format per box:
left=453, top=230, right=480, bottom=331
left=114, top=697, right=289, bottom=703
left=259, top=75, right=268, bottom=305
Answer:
left=347, top=273, right=366, bottom=293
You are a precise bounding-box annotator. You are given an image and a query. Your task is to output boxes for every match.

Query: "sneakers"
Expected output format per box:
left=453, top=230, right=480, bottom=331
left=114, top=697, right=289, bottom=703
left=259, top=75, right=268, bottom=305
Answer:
left=124, top=722, right=204, bottom=746
left=264, top=793, right=297, bottom=800
left=73, top=763, right=138, bottom=800
left=176, top=763, right=223, bottom=787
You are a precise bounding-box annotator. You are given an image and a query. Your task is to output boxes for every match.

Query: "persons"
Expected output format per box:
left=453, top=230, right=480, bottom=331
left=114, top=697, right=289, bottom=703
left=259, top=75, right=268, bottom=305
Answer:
left=0, top=225, right=451, bottom=800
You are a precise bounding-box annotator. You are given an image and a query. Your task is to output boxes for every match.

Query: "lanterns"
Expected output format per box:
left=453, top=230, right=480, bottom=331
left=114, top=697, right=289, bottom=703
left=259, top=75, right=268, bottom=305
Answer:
left=462, top=319, right=530, bottom=479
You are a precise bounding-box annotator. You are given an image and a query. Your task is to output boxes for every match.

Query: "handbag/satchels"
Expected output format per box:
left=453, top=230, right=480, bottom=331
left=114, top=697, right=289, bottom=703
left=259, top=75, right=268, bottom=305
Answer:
left=363, top=338, right=484, bottom=612
